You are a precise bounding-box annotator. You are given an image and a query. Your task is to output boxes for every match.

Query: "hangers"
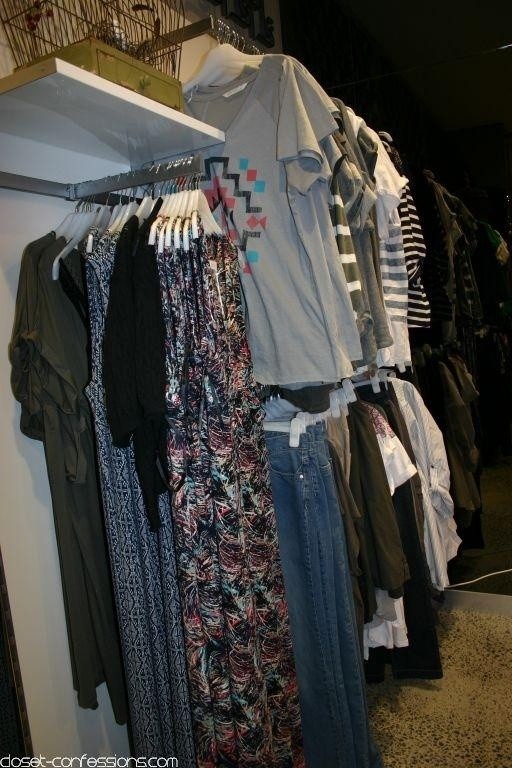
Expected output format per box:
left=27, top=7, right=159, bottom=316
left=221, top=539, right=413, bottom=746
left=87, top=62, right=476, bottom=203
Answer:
left=181, top=18, right=272, bottom=91
left=239, top=367, right=392, bottom=446
left=45, top=157, right=223, bottom=281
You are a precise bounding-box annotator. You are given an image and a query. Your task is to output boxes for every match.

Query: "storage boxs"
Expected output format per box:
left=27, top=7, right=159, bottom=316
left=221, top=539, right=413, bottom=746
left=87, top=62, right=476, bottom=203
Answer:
left=14, top=38, right=183, bottom=113
